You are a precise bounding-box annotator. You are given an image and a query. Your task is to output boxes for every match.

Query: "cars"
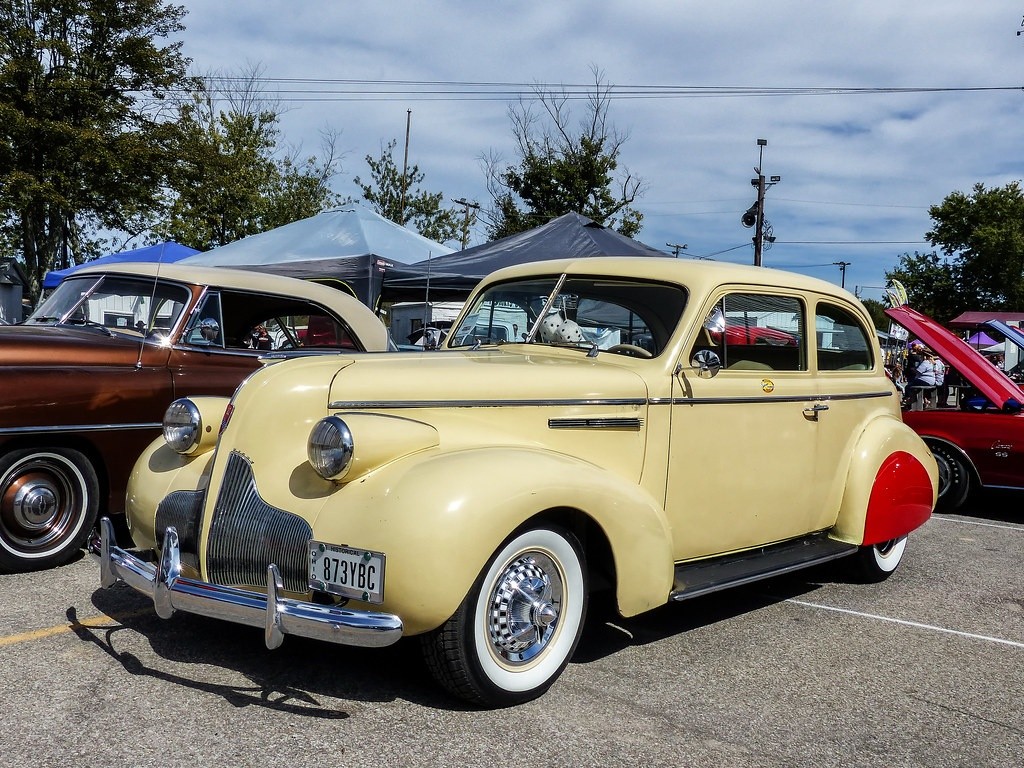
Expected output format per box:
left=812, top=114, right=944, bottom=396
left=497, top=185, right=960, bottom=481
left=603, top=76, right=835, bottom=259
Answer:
left=884, top=305, right=1024, bottom=514
left=101, top=256, right=940, bottom=710
left=1, top=264, right=394, bottom=570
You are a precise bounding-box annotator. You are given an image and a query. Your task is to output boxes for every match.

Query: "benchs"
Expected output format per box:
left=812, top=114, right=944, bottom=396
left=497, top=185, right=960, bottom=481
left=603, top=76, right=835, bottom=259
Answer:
left=948, top=384, right=974, bottom=408
left=690, top=346, right=870, bottom=372
left=911, top=386, right=936, bottom=409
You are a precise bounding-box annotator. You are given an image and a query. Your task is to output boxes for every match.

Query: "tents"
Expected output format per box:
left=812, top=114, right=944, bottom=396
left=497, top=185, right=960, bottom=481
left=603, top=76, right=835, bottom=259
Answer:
left=0, top=201, right=799, bottom=348
left=965, top=331, right=1006, bottom=373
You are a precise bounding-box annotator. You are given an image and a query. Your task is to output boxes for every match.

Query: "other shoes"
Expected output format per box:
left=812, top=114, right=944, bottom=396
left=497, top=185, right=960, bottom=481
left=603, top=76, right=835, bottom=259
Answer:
left=936, top=401, right=949, bottom=408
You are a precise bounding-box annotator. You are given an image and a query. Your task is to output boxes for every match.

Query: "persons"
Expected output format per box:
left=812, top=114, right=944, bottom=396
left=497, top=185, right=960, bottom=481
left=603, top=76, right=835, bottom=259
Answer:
left=888, top=343, right=1006, bottom=411
